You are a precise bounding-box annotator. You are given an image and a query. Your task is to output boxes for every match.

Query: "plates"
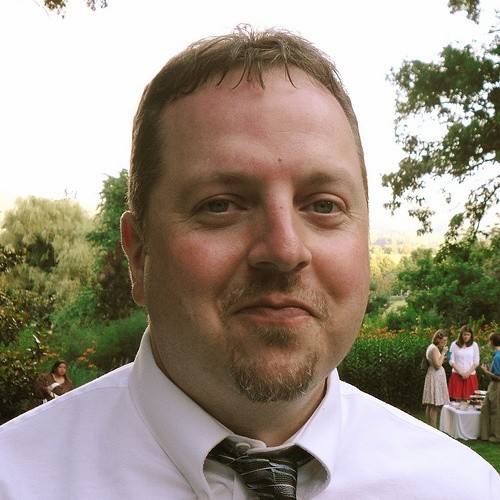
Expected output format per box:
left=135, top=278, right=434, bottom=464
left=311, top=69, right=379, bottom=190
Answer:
left=470, top=389, right=487, bottom=404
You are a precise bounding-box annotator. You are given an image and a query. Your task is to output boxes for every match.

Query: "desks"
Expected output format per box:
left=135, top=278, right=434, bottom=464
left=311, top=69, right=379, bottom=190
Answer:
left=440, top=401, right=481, bottom=441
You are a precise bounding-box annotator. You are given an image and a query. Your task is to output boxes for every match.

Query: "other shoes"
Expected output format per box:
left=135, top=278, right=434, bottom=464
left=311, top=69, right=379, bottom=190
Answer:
left=489, top=437, right=500, bottom=442
left=477, top=437, right=489, bottom=443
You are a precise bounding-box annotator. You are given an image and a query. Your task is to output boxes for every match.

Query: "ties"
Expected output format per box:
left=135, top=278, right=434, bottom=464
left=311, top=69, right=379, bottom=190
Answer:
left=207, top=441, right=314, bottom=500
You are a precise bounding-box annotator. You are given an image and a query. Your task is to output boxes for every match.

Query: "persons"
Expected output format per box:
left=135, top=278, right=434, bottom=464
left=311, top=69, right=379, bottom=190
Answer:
left=422, top=329, right=450, bottom=429
left=479, top=332, right=500, bottom=443
left=447, top=325, right=480, bottom=403
left=0, top=23, right=500, bottom=500
left=28, top=359, right=75, bottom=411
